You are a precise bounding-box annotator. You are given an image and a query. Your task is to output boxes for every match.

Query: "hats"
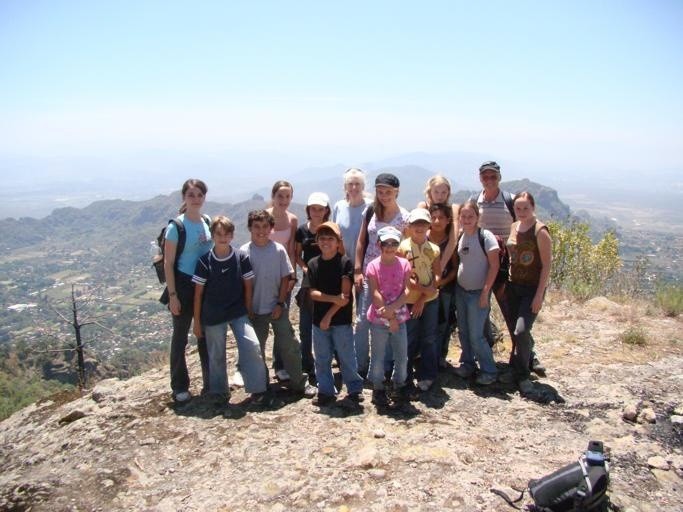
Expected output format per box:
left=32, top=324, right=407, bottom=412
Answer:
left=407, top=207, right=433, bottom=226
left=306, top=192, right=330, bottom=208
left=375, top=172, right=399, bottom=189
left=313, top=221, right=343, bottom=241
left=478, top=159, right=501, bottom=175
left=376, top=226, right=402, bottom=245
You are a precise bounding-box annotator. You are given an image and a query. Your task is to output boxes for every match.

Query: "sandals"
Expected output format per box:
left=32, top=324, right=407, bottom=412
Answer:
left=250, top=393, right=285, bottom=408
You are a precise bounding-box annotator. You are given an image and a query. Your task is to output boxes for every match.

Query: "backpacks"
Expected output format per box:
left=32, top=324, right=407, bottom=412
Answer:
left=150, top=218, right=189, bottom=281
left=478, top=226, right=511, bottom=288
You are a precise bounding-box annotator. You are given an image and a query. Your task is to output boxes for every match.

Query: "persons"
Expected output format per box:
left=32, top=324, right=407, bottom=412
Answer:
left=150, top=161, right=553, bottom=411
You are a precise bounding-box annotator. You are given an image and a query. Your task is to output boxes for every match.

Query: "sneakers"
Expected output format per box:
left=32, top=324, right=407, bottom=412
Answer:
left=452, top=364, right=528, bottom=386
left=172, top=391, right=192, bottom=403
left=394, top=375, right=437, bottom=400
left=274, top=369, right=290, bottom=380
left=531, top=356, right=545, bottom=373
left=370, top=390, right=391, bottom=407
left=231, top=372, right=245, bottom=389
left=303, top=387, right=364, bottom=406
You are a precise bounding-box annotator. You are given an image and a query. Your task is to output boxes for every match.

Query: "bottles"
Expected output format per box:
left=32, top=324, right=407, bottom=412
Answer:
left=587, top=442, right=604, bottom=467
left=148, top=241, right=164, bottom=265
